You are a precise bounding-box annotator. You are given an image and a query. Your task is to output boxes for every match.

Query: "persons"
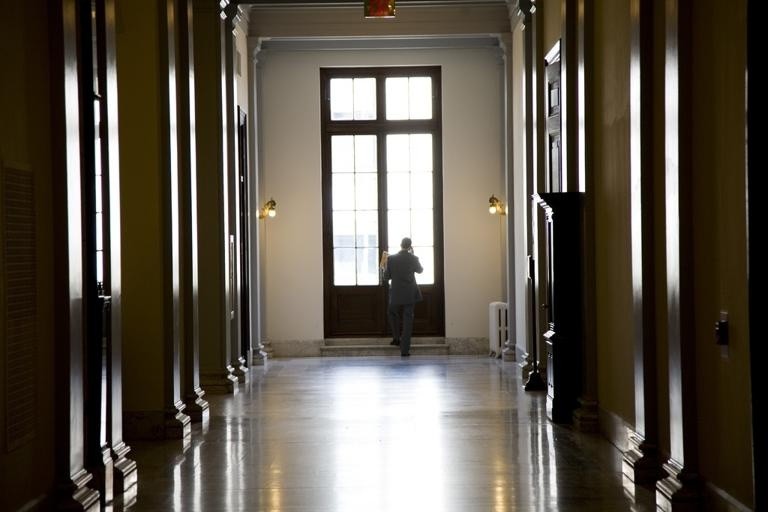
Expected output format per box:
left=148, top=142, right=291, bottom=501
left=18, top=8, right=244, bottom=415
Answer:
left=380, top=237, right=424, bottom=357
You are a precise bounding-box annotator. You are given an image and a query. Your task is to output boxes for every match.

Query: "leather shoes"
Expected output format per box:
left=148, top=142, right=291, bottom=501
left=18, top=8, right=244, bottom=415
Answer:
left=401, top=353, right=410, bottom=356
left=390, top=340, right=400, bottom=345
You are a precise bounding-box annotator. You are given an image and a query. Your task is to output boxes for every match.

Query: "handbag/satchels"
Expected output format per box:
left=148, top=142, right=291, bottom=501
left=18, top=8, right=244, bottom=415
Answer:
left=380, top=251, right=390, bottom=271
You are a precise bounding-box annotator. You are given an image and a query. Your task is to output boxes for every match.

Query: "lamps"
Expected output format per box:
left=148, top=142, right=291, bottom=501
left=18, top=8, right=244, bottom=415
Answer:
left=487, top=193, right=503, bottom=216
left=260, top=196, right=278, bottom=220
